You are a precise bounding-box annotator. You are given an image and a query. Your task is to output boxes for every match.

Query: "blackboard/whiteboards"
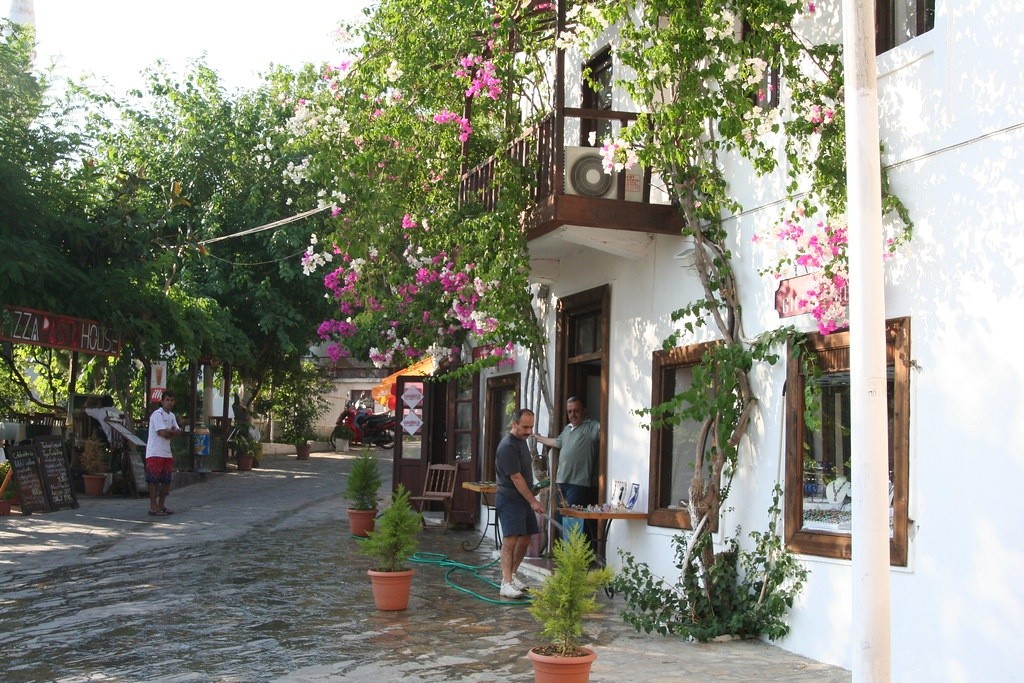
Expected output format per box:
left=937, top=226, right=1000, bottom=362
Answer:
left=35, top=434, right=79, bottom=507
left=5, top=444, right=51, bottom=511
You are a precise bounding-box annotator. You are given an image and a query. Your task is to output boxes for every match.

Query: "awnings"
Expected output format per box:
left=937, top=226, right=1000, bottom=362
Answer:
left=371, top=357, right=434, bottom=406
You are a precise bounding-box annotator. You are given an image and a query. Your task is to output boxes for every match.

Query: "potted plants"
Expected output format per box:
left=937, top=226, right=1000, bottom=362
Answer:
left=353, top=482, right=424, bottom=611
left=525, top=521, right=617, bottom=683
left=344, top=445, right=382, bottom=538
left=0, top=460, right=19, bottom=515
left=334, top=425, right=356, bottom=453
left=294, top=437, right=312, bottom=459
left=81, top=431, right=112, bottom=495
left=231, top=421, right=255, bottom=471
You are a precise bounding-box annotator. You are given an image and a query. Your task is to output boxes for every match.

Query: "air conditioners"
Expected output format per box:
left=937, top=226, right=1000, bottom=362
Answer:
left=562, top=147, right=644, bottom=203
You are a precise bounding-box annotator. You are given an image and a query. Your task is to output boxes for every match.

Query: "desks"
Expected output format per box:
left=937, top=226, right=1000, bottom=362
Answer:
left=560, top=508, right=648, bottom=599
left=461, top=482, right=502, bottom=552
left=0, top=423, right=63, bottom=444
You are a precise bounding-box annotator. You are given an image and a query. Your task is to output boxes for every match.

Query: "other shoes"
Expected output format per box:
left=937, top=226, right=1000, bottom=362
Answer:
left=149, top=510, right=167, bottom=516
left=158, top=507, right=173, bottom=514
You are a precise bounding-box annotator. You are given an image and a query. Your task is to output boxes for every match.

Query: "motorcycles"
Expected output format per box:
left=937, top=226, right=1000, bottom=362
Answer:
left=330, top=390, right=396, bottom=450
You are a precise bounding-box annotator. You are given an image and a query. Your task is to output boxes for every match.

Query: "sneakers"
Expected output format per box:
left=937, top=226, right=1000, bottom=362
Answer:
left=500, top=582, right=523, bottom=599
left=512, top=573, right=529, bottom=591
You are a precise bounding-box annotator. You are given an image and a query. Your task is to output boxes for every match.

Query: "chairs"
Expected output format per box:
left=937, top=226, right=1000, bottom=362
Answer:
left=180, top=415, right=234, bottom=432
left=0, top=411, right=67, bottom=447
left=410, top=462, right=459, bottom=535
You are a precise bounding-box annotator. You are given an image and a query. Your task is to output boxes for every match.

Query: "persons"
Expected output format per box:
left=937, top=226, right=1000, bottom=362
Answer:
left=144, top=391, right=183, bottom=515
left=531, top=396, right=602, bottom=542
left=79, top=395, right=123, bottom=446
left=495, top=408, right=544, bottom=597
